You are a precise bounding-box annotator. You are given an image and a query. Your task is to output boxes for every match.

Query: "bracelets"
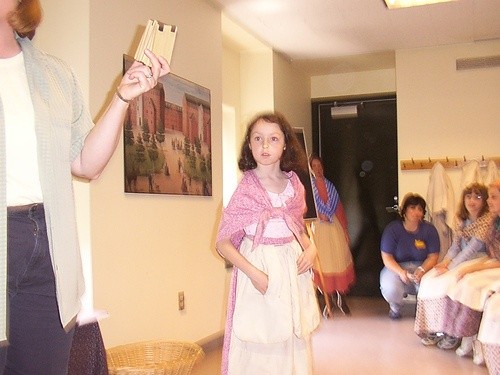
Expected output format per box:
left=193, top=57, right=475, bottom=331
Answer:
left=116, top=89, right=130, bottom=103
left=418, top=266, right=426, bottom=273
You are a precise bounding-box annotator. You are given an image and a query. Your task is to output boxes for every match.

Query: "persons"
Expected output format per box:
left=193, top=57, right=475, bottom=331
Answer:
left=301, top=157, right=357, bottom=314
left=380, top=192, right=440, bottom=320
left=0, top=1, right=170, bottom=375
left=415, top=182, right=500, bottom=375
left=216, top=110, right=329, bottom=375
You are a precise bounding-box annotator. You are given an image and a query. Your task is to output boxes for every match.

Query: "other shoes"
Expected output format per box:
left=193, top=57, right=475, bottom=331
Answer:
left=336, top=298, right=351, bottom=317
left=456, top=335, right=474, bottom=356
left=387, top=308, right=401, bottom=321
left=421, top=333, right=445, bottom=346
left=436, top=335, right=460, bottom=350
left=323, top=300, right=334, bottom=321
left=472, top=338, right=485, bottom=365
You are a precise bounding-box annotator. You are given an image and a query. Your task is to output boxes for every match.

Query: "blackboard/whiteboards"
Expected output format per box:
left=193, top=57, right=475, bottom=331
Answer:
left=281, top=127, right=319, bottom=222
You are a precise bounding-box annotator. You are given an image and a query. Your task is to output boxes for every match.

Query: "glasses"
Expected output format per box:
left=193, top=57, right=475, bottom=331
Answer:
left=465, top=194, right=483, bottom=200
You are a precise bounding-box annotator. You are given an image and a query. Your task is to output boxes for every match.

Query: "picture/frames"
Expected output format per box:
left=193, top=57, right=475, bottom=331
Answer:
left=122, top=52, right=213, bottom=199
